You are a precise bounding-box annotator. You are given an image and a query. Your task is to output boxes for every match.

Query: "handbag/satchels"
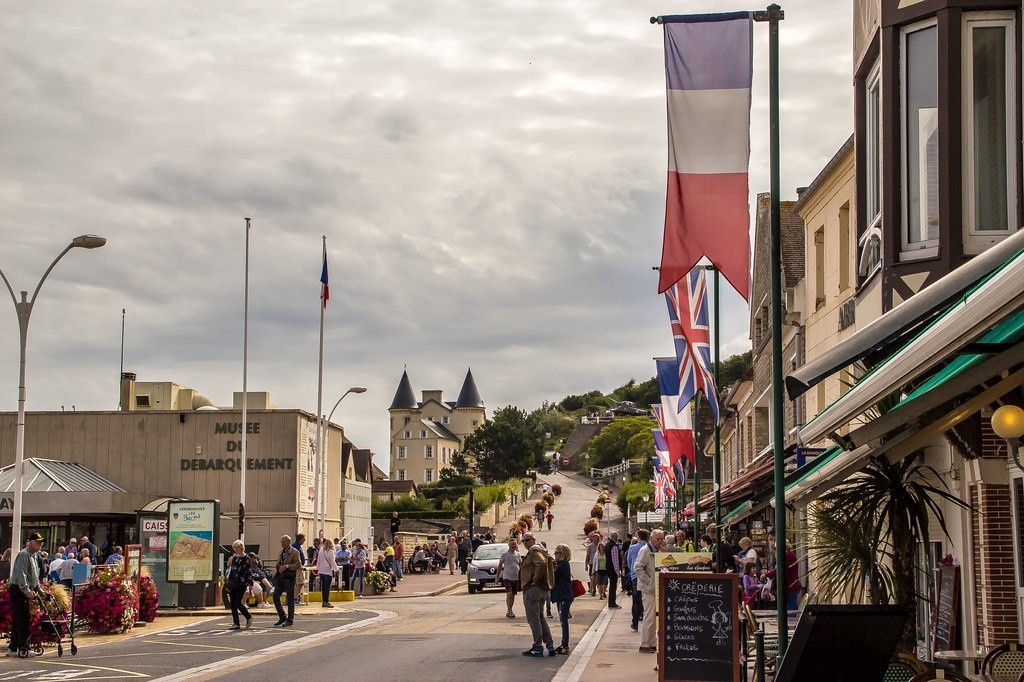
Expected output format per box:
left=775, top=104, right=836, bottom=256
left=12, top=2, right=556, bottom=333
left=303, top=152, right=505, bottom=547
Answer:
left=571, top=579, right=586, bottom=597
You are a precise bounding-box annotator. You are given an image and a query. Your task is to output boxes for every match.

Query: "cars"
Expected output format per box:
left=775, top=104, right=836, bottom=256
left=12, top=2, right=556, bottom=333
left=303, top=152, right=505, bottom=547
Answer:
left=465, top=543, right=509, bottom=594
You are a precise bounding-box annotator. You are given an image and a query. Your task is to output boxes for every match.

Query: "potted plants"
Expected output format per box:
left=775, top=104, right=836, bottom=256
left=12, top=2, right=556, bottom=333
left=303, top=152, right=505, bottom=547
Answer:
left=584, top=491, right=609, bottom=546
left=509, top=484, right=562, bottom=542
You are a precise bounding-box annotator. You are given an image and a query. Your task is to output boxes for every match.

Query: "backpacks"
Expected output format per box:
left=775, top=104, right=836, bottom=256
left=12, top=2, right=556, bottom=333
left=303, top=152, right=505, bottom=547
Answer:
left=533, top=550, right=556, bottom=590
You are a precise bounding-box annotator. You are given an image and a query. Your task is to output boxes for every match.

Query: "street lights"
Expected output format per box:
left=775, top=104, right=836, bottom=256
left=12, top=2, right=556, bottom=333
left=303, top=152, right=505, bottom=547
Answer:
left=0, top=233, right=108, bottom=581
left=320, top=387, right=368, bottom=536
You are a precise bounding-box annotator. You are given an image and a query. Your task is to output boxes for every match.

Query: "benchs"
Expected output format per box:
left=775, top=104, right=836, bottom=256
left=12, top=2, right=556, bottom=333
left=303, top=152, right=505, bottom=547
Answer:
left=412, top=562, right=425, bottom=575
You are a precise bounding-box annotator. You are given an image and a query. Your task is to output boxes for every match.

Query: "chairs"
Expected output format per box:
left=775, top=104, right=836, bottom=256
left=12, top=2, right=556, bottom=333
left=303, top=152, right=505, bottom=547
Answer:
left=733, top=600, right=1024, bottom=682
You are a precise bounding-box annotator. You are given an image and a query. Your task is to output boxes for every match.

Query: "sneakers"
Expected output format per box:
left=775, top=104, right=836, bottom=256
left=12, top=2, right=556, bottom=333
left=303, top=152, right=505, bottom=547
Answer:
left=523, top=649, right=544, bottom=658
left=548, top=648, right=556, bottom=656
left=631, top=623, right=638, bottom=632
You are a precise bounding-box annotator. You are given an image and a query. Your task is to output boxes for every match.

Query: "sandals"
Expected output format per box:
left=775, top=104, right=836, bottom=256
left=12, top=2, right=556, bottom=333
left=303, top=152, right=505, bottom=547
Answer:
left=508, top=612, right=515, bottom=619
left=556, top=646, right=569, bottom=655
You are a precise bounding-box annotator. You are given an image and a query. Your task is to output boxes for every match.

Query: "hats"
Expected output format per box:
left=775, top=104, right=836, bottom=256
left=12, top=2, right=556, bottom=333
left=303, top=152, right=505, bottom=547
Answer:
left=28, top=533, right=44, bottom=541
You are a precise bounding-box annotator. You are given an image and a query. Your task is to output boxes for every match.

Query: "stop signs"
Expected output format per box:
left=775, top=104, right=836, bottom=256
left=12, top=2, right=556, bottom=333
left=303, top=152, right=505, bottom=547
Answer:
left=563, top=457, right=569, bottom=465
left=606, top=497, right=611, bottom=503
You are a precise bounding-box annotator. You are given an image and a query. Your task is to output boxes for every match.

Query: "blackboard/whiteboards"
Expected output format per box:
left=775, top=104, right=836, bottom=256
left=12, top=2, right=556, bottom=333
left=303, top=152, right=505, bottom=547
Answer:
left=658, top=572, right=741, bottom=682
left=935, top=565, right=959, bottom=644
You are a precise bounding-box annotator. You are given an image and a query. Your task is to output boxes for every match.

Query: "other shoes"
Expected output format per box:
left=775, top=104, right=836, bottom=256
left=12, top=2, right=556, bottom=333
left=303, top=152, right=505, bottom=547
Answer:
left=282, top=620, right=294, bottom=627
left=229, top=621, right=240, bottom=630
left=609, top=604, right=621, bottom=609
left=273, top=617, right=287, bottom=626
left=639, top=647, right=655, bottom=653
left=246, top=616, right=253, bottom=628
left=20, top=651, right=36, bottom=658
left=322, top=602, right=334, bottom=608
left=7, top=649, right=19, bottom=657
left=390, top=589, right=398, bottom=592
left=600, top=593, right=607, bottom=599
left=359, top=594, right=363, bottom=598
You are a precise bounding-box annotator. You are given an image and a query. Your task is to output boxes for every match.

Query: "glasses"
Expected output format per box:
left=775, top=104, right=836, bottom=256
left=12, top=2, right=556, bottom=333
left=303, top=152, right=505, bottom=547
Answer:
left=521, top=539, right=532, bottom=542
left=71, top=542, right=77, bottom=544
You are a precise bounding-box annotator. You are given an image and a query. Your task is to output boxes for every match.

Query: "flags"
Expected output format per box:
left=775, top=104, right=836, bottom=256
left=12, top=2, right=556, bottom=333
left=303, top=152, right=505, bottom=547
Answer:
left=320, top=245, right=329, bottom=310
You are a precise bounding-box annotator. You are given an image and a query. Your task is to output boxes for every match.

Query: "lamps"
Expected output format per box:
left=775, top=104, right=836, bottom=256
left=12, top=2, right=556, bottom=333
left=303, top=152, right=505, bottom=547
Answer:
left=991, top=405, right=1024, bottom=471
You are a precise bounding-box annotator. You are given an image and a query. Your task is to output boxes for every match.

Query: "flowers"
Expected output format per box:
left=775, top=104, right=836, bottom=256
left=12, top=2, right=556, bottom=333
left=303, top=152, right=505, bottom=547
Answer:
left=0, top=569, right=157, bottom=645
left=365, top=570, right=395, bottom=594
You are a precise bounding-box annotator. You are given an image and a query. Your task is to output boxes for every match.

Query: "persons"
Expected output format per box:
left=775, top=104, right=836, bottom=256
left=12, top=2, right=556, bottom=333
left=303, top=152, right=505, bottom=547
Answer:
left=586, top=524, right=800, bottom=635
left=0, top=535, right=126, bottom=596
left=226, top=539, right=254, bottom=630
left=634, top=530, right=665, bottom=654
left=553, top=452, right=558, bottom=475
left=225, top=533, right=404, bottom=611
left=538, top=510, right=554, bottom=532
left=412, top=530, right=500, bottom=576
left=7, top=533, right=49, bottom=659
left=647, top=410, right=655, bottom=422
left=272, top=535, right=301, bottom=627
left=390, top=512, right=401, bottom=545
left=542, top=483, right=548, bottom=496
left=587, top=408, right=610, bottom=424
left=521, top=532, right=556, bottom=657
left=498, top=538, right=573, bottom=618
left=552, top=544, right=573, bottom=655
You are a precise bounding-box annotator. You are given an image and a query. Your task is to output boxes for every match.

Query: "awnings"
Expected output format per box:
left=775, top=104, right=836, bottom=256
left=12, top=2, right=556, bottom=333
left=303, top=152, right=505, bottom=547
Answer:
left=686, top=438, right=894, bottom=530
left=784, top=226, right=1024, bottom=463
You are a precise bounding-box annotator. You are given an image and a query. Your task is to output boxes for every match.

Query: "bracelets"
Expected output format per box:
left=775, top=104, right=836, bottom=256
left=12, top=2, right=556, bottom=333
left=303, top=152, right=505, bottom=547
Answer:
left=285, top=565, right=288, bottom=569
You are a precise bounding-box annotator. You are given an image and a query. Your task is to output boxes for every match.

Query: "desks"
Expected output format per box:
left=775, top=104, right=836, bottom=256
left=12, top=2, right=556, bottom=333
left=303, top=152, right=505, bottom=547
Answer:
left=741, top=609, right=800, bottom=632
left=934, top=650, right=997, bottom=674
